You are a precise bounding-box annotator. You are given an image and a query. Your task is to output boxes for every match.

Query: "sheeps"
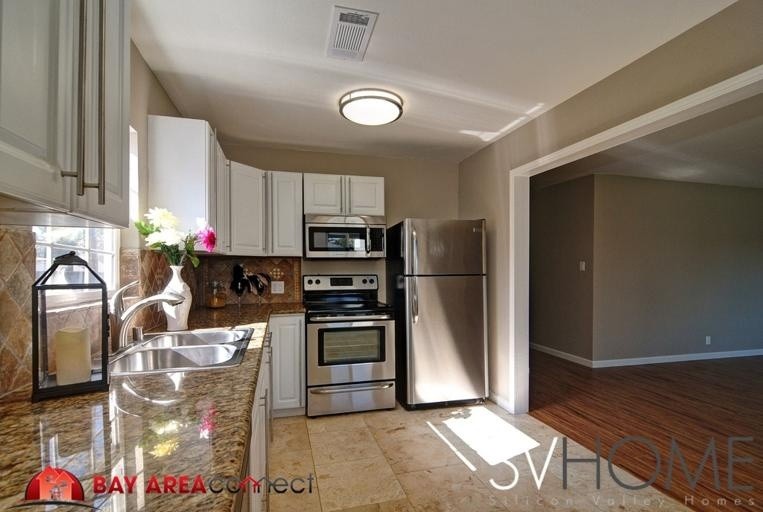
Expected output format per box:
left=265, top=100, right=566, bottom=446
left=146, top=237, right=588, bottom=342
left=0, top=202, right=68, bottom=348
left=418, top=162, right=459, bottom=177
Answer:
left=107, top=376, right=185, bottom=419
left=108, top=280, right=186, bottom=351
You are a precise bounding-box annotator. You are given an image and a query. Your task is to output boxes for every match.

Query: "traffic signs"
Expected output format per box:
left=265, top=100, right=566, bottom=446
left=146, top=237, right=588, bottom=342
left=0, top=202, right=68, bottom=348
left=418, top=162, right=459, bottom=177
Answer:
left=271, top=280, right=284, bottom=294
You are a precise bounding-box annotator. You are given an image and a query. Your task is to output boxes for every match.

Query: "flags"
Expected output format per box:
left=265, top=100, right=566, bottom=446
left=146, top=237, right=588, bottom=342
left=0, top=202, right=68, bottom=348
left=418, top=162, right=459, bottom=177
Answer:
left=163, top=265, right=192, bottom=332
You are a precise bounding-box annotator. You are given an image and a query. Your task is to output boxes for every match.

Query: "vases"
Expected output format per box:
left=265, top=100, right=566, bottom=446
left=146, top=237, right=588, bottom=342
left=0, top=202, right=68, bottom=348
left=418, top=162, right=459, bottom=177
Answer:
left=135, top=206, right=217, bottom=270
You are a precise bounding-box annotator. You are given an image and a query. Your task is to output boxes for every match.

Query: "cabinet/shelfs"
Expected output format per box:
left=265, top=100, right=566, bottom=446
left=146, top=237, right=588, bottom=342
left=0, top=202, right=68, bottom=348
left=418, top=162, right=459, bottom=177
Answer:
left=205, top=280, right=226, bottom=308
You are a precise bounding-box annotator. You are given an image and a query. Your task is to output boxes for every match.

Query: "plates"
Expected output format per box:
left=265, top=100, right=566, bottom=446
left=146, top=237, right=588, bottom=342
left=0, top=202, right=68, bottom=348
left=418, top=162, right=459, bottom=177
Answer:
left=0, top=0, right=133, bottom=226
left=147, top=114, right=385, bottom=258
left=242, top=313, right=307, bottom=512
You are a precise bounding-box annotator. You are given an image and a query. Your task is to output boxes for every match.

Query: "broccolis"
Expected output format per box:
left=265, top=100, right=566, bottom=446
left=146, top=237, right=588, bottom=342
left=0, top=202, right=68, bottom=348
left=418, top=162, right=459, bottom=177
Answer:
left=387, top=218, right=490, bottom=411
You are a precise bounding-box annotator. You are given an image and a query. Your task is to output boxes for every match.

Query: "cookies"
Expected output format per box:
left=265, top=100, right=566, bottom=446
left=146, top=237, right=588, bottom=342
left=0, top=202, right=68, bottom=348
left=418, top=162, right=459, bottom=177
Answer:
left=305, top=223, right=386, bottom=258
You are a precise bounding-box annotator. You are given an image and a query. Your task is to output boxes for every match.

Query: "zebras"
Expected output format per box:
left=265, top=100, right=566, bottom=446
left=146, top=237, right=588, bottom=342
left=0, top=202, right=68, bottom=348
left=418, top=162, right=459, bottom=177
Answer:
left=306, top=314, right=396, bottom=417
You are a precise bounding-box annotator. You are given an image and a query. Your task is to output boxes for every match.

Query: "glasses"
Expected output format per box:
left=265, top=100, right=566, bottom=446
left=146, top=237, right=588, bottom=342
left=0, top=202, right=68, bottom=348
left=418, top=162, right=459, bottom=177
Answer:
left=338, top=87, right=404, bottom=126
